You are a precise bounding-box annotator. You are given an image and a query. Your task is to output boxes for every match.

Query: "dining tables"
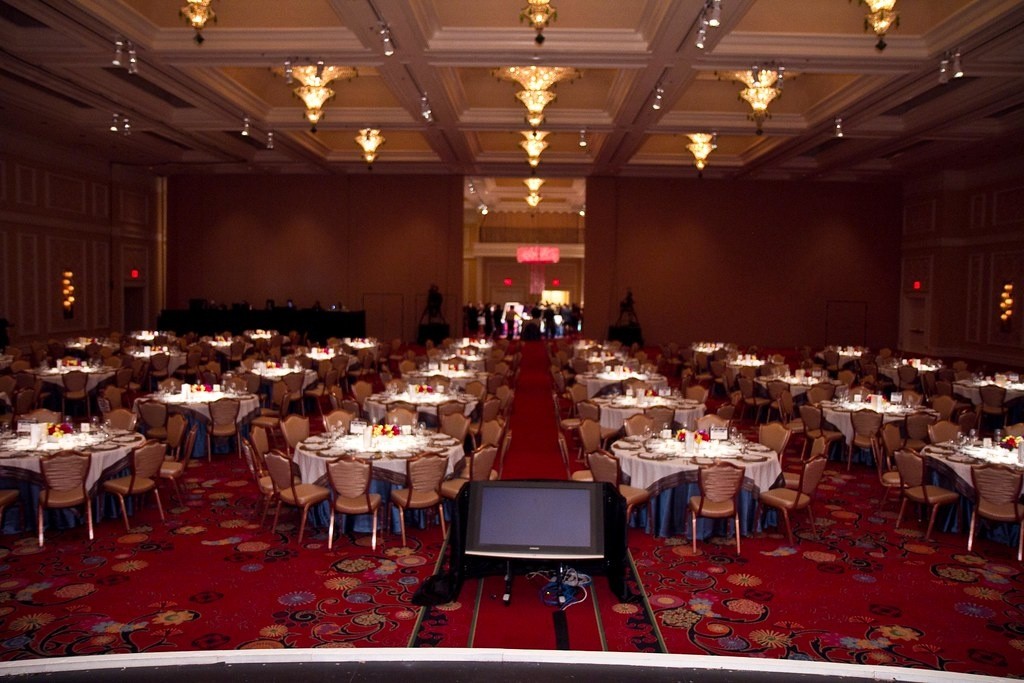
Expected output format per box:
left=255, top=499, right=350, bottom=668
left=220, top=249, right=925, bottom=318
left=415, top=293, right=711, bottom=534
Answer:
left=691, top=344, right=725, bottom=367
left=409, top=369, right=487, bottom=390
left=590, top=396, right=707, bottom=431
left=64, top=341, right=120, bottom=355
left=365, top=391, right=477, bottom=423
left=611, top=432, right=786, bottom=540
left=125, top=349, right=187, bottom=370
left=251, top=333, right=272, bottom=341
left=920, top=438, right=1024, bottom=549
left=755, top=375, right=826, bottom=402
left=293, top=426, right=465, bottom=529
left=434, top=354, right=484, bottom=371
left=953, top=379, right=1024, bottom=415
left=344, top=342, right=376, bottom=351
left=449, top=341, right=491, bottom=353
left=728, top=359, right=766, bottom=383
left=133, top=334, right=170, bottom=343
left=135, top=391, right=261, bottom=433
left=575, top=371, right=668, bottom=396
left=286, top=352, right=350, bottom=368
left=568, top=344, right=595, bottom=351
left=569, top=356, right=620, bottom=371
left=0, top=426, right=146, bottom=532
left=816, top=396, right=918, bottom=447
left=24, top=364, right=116, bottom=396
left=238, top=361, right=318, bottom=394
left=878, top=362, right=944, bottom=389
left=208, top=340, right=253, bottom=355
left=817, top=350, right=862, bottom=370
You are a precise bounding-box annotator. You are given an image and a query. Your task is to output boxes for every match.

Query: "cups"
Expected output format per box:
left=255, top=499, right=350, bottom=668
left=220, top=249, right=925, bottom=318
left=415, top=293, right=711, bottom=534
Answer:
left=663, top=423, right=669, bottom=428
left=1017, top=442, right=1024, bottom=464
left=652, top=432, right=658, bottom=445
left=682, top=422, right=688, bottom=430
left=994, top=430, right=1002, bottom=443
left=2, top=422, right=10, bottom=434
left=971, top=429, right=978, bottom=445
left=645, top=426, right=651, bottom=437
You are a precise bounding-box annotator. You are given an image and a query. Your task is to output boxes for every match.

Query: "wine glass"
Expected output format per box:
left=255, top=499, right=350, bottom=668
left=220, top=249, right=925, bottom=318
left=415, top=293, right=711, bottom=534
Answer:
left=957, top=432, right=967, bottom=449
left=729, top=427, right=736, bottom=446
left=735, top=433, right=743, bottom=446
left=62, top=414, right=111, bottom=440
left=411, top=418, right=426, bottom=444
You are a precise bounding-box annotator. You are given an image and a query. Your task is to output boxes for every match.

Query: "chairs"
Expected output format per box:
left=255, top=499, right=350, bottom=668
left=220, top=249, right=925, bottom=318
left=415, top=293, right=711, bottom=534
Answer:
left=0, top=329, right=1024, bottom=564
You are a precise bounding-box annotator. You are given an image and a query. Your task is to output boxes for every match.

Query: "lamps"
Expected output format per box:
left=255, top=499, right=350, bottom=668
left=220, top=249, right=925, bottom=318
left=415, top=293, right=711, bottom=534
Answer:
left=654, top=87, right=664, bottom=110
left=109, top=114, right=132, bottom=138
left=421, top=96, right=435, bottom=126
left=354, top=128, right=385, bottom=167
left=937, top=58, right=949, bottom=83
left=112, top=38, right=138, bottom=74
left=951, top=52, right=964, bottom=79
left=849, top=0, right=900, bottom=50
left=380, top=21, right=394, bottom=56
left=242, top=117, right=251, bottom=136
left=179, top=0, right=217, bottom=45
left=733, top=66, right=785, bottom=137
left=490, top=0, right=587, bottom=218
left=835, top=118, right=843, bottom=138
left=696, top=0, right=723, bottom=49
left=685, top=133, right=718, bottom=170
left=270, top=59, right=358, bottom=135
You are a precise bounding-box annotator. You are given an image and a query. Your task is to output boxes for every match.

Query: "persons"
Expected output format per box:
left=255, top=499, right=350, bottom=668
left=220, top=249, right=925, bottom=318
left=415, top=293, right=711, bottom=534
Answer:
left=542, top=304, right=557, bottom=339
left=479, top=303, right=492, bottom=338
left=558, top=303, right=581, bottom=338
left=464, top=301, right=480, bottom=338
left=505, top=305, right=526, bottom=342
left=530, top=302, right=542, bottom=319
left=286, top=299, right=297, bottom=308
left=492, top=304, right=504, bottom=338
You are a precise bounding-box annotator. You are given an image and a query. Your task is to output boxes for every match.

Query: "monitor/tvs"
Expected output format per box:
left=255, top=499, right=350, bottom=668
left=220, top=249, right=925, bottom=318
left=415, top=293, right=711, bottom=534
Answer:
left=463, top=480, right=605, bottom=560
